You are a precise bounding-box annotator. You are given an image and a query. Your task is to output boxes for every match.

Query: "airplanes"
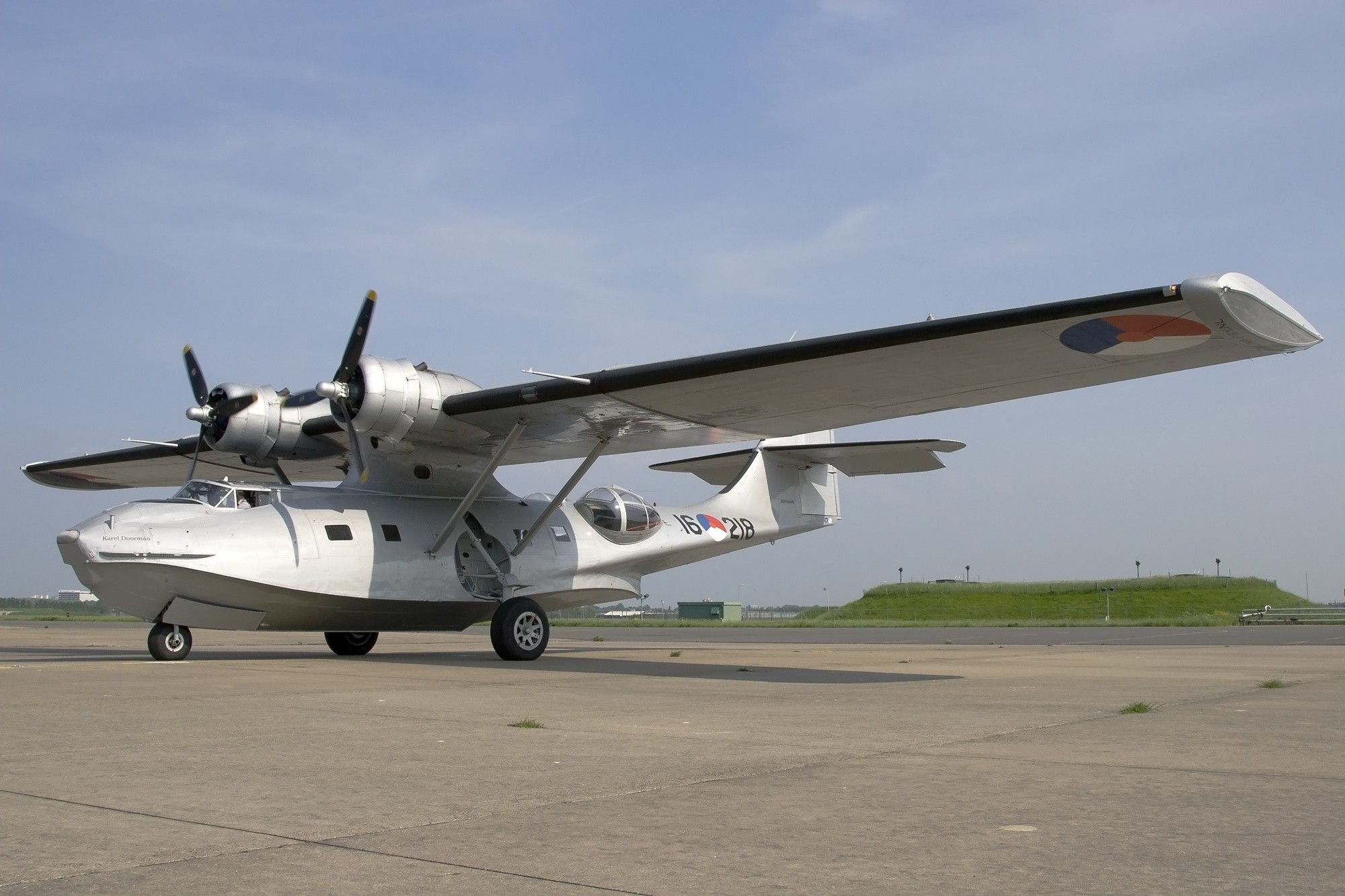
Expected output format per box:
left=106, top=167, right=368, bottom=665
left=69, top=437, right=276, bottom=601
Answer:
left=20, top=270, right=1327, bottom=662
